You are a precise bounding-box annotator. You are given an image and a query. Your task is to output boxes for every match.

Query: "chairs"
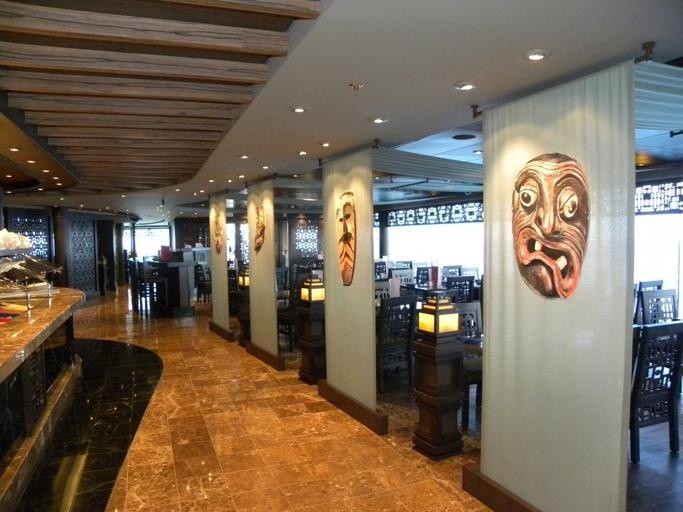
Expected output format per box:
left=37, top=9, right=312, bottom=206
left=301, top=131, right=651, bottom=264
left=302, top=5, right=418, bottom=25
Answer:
left=394, top=260, right=412, bottom=269
left=374, top=295, right=416, bottom=395
left=392, top=270, right=415, bottom=283
left=446, top=276, right=473, bottom=303
left=416, top=267, right=429, bottom=284
left=194, top=264, right=211, bottom=303
left=629, top=320, right=682, bottom=463
left=129, top=261, right=168, bottom=318
left=640, top=290, right=678, bottom=324
left=450, top=302, right=483, bottom=337
left=374, top=261, right=385, bottom=277
left=634, top=280, right=663, bottom=323
left=441, top=266, right=461, bottom=284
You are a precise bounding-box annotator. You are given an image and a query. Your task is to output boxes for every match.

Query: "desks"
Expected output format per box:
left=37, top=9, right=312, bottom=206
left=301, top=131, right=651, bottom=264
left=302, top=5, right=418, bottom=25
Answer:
left=461, top=338, right=473, bottom=430
left=128, top=256, right=195, bottom=319
left=402, top=282, right=448, bottom=297
left=0, top=286, right=86, bottom=511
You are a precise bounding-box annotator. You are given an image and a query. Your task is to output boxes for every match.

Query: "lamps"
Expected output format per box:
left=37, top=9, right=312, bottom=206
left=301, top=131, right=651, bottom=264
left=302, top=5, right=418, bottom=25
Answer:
left=235, top=263, right=250, bottom=347
left=297, top=274, right=325, bottom=385
left=413, top=290, right=465, bottom=462
